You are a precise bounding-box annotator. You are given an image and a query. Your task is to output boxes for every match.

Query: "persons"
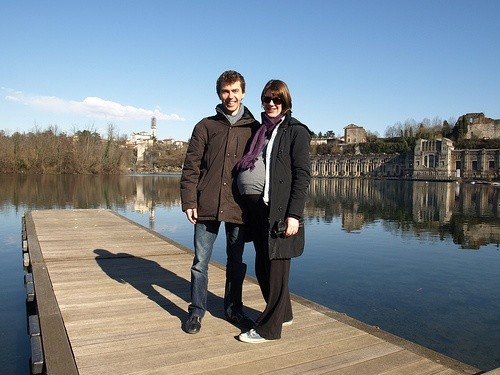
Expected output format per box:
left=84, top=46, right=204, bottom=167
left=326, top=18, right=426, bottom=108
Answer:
left=237, top=80, right=312, bottom=343
left=179, top=70, right=261, bottom=334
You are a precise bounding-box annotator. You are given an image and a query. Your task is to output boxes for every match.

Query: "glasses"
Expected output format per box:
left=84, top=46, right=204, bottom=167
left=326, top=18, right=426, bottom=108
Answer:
left=262, top=96, right=283, bottom=104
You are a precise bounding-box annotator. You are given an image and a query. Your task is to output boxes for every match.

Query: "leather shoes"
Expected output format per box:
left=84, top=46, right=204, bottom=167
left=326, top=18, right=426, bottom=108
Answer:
left=225, top=312, right=255, bottom=330
left=184, top=315, right=204, bottom=333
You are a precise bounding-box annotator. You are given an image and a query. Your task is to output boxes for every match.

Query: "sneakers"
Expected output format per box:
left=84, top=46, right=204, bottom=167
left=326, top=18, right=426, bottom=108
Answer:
left=282, top=319, right=293, bottom=327
left=238, top=329, right=279, bottom=344
